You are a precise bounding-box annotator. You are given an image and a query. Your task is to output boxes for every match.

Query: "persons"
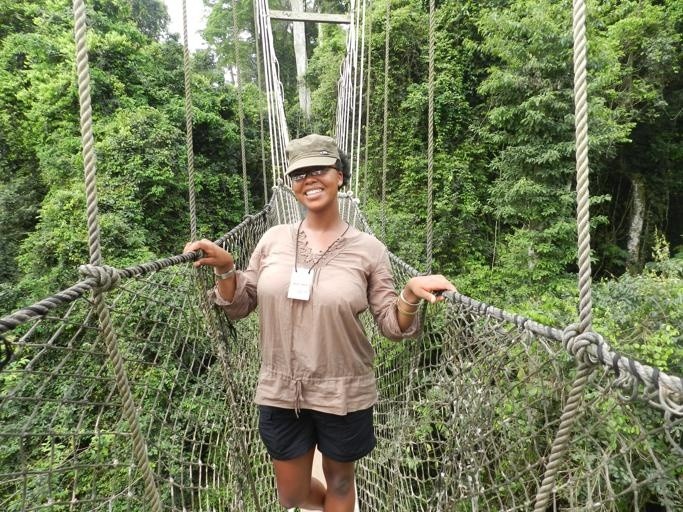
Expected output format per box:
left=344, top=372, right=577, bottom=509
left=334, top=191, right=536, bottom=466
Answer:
left=183, top=133, right=462, bottom=512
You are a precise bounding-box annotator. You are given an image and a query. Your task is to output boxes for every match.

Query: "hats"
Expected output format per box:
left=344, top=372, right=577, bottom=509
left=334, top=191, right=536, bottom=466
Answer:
left=285, top=134, right=341, bottom=175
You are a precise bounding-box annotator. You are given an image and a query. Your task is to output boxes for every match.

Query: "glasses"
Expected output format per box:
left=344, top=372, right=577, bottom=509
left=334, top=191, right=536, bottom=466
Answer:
left=291, top=165, right=339, bottom=180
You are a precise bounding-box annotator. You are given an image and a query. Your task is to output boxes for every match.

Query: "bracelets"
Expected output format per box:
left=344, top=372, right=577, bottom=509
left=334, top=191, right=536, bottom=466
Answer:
left=214, top=263, right=237, bottom=283
left=394, top=299, right=421, bottom=317
left=399, top=290, right=424, bottom=308
